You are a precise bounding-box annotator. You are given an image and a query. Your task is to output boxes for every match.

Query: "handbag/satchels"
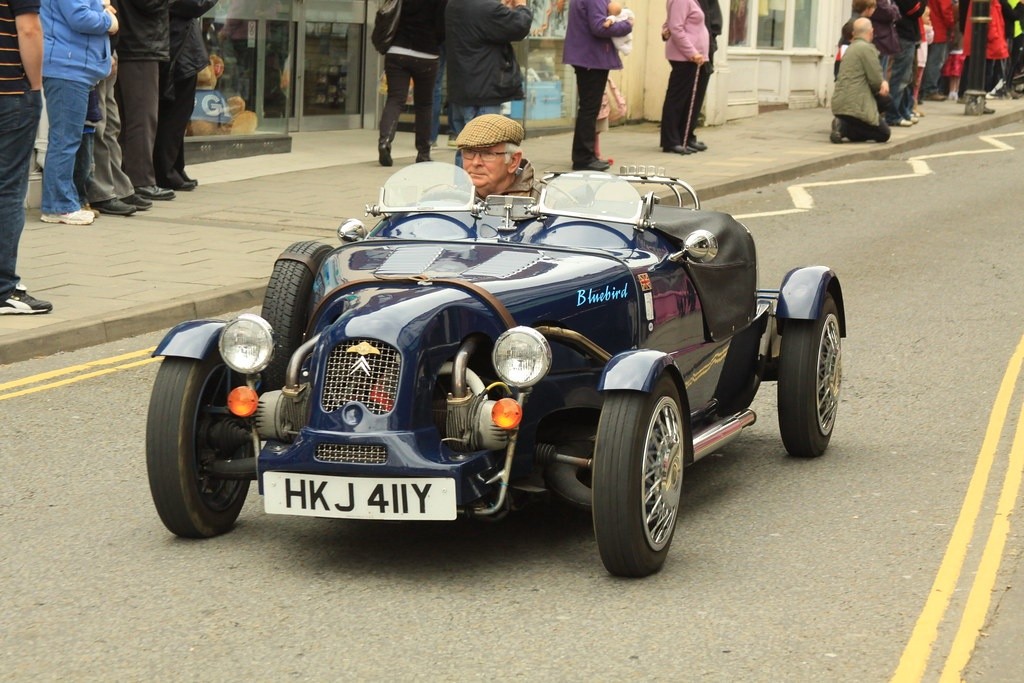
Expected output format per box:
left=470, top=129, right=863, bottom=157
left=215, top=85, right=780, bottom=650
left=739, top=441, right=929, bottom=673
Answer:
left=371, top=0, right=402, bottom=55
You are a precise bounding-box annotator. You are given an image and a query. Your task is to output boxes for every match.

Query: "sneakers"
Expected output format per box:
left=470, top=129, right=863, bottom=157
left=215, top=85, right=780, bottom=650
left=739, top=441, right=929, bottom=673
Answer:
left=0, top=284, right=52, bottom=314
left=41, top=207, right=93, bottom=224
left=77, top=209, right=95, bottom=218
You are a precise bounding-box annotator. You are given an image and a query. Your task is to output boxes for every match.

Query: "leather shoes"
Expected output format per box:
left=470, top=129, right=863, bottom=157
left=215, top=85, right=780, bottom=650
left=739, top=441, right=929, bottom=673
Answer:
left=121, top=195, right=153, bottom=210
left=572, top=159, right=611, bottom=170
left=158, top=179, right=197, bottom=191
left=89, top=197, right=136, bottom=214
left=133, top=185, right=175, bottom=200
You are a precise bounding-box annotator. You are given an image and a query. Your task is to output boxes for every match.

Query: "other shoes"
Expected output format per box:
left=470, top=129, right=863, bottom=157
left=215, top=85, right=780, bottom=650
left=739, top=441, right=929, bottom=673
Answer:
left=416, top=152, right=432, bottom=164
left=688, top=142, right=707, bottom=151
left=883, top=84, right=1024, bottom=126
left=378, top=144, right=393, bottom=167
left=830, top=118, right=846, bottom=143
left=663, top=145, right=691, bottom=154
left=682, top=145, right=697, bottom=152
left=81, top=206, right=100, bottom=217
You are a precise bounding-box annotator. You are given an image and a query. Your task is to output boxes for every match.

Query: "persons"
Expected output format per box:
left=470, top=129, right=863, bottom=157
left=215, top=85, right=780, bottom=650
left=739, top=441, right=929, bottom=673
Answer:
left=378, top=0, right=534, bottom=188
left=834, top=0, right=1024, bottom=127
left=435, top=114, right=561, bottom=207
left=561, top=0, right=636, bottom=171
left=218, top=0, right=289, bottom=117
left=660, top=0, right=723, bottom=154
left=830, top=17, right=891, bottom=143
left=0, top=0, right=217, bottom=317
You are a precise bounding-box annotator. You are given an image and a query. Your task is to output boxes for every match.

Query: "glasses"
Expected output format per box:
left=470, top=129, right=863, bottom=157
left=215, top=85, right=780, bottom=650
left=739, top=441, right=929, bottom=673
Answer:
left=460, top=148, right=512, bottom=161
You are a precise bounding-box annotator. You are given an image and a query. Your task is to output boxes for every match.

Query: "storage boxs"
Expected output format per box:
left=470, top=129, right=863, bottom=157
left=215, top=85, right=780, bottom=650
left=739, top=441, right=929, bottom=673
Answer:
left=511, top=80, right=562, bottom=119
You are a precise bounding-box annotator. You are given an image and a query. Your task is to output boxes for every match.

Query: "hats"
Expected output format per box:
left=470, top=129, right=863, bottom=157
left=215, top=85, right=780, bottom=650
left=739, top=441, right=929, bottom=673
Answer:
left=456, top=114, right=523, bottom=145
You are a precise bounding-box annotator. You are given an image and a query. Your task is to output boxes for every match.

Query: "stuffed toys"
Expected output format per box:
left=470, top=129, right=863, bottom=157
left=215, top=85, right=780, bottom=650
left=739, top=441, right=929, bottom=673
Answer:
left=185, top=56, right=257, bottom=135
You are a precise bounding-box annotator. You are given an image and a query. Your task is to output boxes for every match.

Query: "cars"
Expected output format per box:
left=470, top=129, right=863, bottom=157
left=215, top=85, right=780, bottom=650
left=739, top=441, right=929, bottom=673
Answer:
left=146, top=161, right=845, bottom=581
